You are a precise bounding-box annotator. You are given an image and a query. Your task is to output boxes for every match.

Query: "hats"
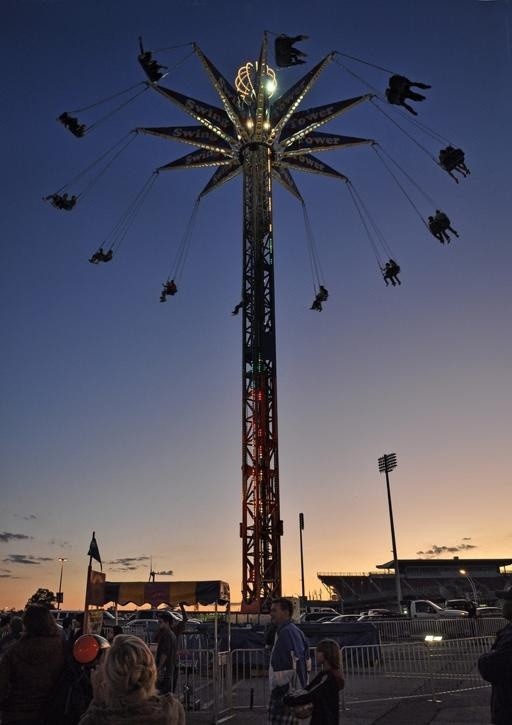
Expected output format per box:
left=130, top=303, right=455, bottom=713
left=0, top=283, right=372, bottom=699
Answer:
left=495, top=586, right=511, bottom=600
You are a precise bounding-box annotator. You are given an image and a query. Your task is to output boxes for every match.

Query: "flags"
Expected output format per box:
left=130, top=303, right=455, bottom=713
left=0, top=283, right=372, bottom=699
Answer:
left=87, top=538, right=102, bottom=572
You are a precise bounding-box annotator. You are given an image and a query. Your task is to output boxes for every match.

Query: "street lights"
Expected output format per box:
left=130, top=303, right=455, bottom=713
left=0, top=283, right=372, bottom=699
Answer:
left=458, top=569, right=480, bottom=602
left=57, top=558, right=68, bottom=610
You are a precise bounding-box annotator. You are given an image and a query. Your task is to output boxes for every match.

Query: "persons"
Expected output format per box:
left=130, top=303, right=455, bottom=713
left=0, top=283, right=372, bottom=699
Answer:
left=88, top=247, right=113, bottom=264
left=385, top=75, right=432, bottom=116
left=140, top=51, right=169, bottom=83
left=379, top=258, right=402, bottom=288
left=427, top=208, right=460, bottom=245
left=230, top=293, right=250, bottom=315
left=267, top=597, right=311, bottom=725
left=60, top=113, right=87, bottom=135
left=280, top=33, right=311, bottom=66
left=159, top=280, right=177, bottom=302
left=477, top=587, right=512, bottom=725
left=308, top=284, right=330, bottom=313
left=41, top=191, right=77, bottom=211
left=439, top=145, right=471, bottom=184
left=1, top=602, right=185, bottom=725
left=276, top=639, right=345, bottom=725
